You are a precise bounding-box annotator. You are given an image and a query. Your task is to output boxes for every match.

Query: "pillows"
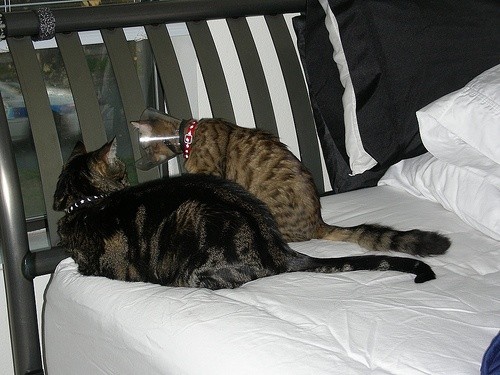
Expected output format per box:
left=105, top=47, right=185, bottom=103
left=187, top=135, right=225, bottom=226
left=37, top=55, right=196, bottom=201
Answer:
left=414, top=64, right=500, bottom=180
left=378, top=152, right=500, bottom=242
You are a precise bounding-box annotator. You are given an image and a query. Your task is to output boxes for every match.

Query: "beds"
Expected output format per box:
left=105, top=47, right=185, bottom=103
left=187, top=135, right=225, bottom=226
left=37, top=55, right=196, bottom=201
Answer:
left=42, top=185, right=500, bottom=375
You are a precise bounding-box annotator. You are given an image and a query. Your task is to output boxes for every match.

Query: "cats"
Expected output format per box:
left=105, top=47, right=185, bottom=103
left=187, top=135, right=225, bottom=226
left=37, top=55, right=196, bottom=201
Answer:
left=129, top=117, right=451, bottom=256
left=52, top=134, right=437, bottom=291
